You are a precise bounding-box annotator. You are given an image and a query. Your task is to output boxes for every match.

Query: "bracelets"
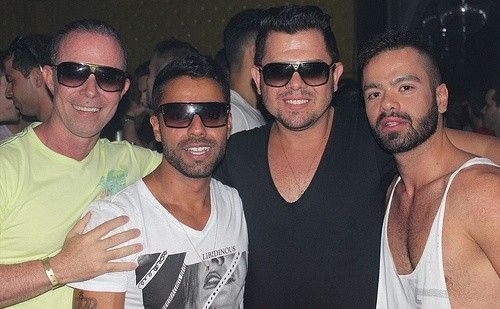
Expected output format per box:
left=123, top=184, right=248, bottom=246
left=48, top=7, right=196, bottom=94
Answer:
left=41, top=257, right=62, bottom=290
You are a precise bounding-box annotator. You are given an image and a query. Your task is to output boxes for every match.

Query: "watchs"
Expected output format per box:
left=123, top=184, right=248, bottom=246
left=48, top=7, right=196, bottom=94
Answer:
left=119, top=114, right=136, bottom=124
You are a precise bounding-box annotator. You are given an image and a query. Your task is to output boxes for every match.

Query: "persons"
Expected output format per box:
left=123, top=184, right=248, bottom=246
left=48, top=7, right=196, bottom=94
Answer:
left=71, top=53, right=250, bottom=309
left=0, top=8, right=273, bottom=154
left=358, top=28, right=500, bottom=309
left=212, top=3, right=500, bottom=309
left=0, top=17, right=164, bottom=309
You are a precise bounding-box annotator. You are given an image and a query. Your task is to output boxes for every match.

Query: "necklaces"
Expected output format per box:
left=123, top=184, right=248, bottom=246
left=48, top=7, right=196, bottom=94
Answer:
left=275, top=121, right=330, bottom=197
left=154, top=169, right=220, bottom=271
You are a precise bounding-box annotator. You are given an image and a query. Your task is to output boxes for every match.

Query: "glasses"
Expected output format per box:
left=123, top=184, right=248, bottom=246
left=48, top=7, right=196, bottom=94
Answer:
left=259, top=60, right=335, bottom=87
left=155, top=102, right=231, bottom=128
left=16, top=33, right=43, bottom=73
left=50, top=61, right=127, bottom=92
